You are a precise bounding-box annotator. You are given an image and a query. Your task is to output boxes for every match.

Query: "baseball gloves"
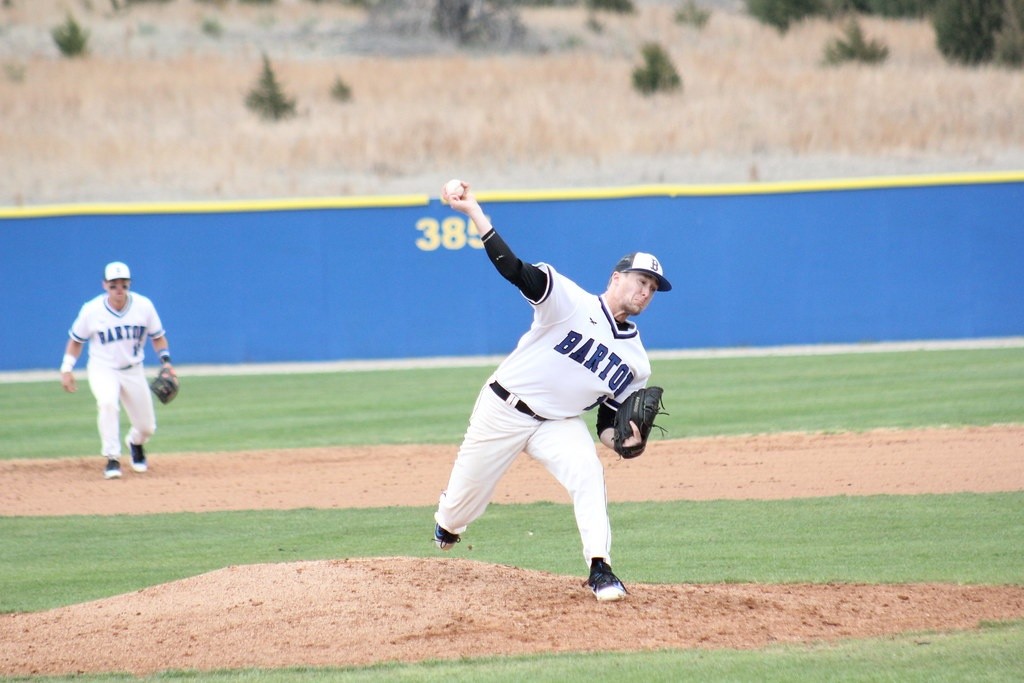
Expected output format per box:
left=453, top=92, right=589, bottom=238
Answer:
left=613, top=385, right=664, bottom=460
left=150, top=367, right=180, bottom=405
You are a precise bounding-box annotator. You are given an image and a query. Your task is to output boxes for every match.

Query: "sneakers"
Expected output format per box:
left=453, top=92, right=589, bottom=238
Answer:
left=588, top=557, right=628, bottom=602
left=124, top=436, right=148, bottom=472
left=431, top=519, right=461, bottom=551
left=104, top=457, right=122, bottom=480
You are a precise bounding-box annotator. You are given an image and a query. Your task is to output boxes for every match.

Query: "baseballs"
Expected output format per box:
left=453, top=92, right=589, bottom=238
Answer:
left=444, top=179, right=465, bottom=199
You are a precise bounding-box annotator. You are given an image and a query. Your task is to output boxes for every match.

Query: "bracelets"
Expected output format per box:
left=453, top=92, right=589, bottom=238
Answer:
left=157, top=349, right=170, bottom=361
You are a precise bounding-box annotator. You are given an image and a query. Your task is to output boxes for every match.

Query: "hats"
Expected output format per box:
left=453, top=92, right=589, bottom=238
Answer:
left=611, top=252, right=672, bottom=292
left=104, top=261, right=130, bottom=281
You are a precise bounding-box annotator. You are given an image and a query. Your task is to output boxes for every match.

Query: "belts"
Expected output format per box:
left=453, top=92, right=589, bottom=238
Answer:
left=489, top=380, right=548, bottom=421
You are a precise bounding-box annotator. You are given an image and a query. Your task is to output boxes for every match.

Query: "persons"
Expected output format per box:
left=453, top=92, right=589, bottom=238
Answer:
left=61, top=262, right=179, bottom=479
left=433, top=180, right=671, bottom=601
